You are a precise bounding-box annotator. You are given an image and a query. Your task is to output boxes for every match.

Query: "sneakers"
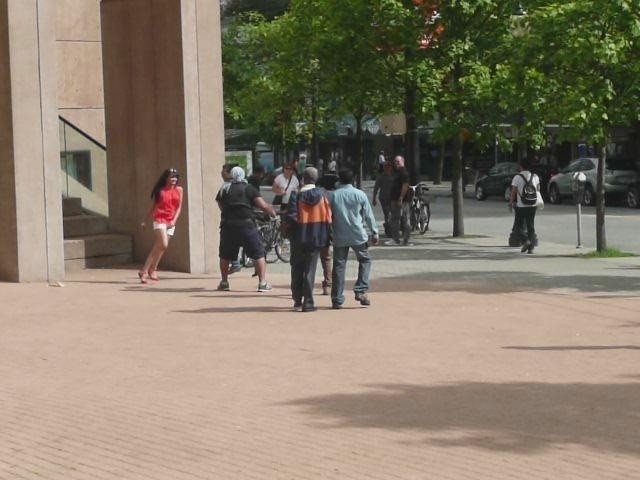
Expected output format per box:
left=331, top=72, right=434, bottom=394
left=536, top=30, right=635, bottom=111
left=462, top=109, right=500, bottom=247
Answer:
left=227, top=266, right=241, bottom=274
left=527, top=249, right=534, bottom=254
left=217, top=282, right=230, bottom=290
left=384, top=240, right=400, bottom=246
left=288, top=283, right=369, bottom=311
left=258, top=282, right=272, bottom=291
left=403, top=241, right=414, bottom=247
left=521, top=240, right=531, bottom=251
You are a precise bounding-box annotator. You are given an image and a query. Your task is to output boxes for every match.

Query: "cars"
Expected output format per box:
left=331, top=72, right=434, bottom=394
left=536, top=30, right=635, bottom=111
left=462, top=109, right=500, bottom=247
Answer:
left=547, top=157, right=640, bottom=207
left=474, top=159, right=558, bottom=203
left=272, top=163, right=340, bottom=189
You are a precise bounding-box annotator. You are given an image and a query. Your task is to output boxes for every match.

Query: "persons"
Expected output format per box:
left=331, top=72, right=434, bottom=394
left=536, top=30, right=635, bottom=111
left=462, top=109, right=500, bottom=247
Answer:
left=508, top=158, right=545, bottom=254
left=215, top=148, right=412, bottom=312
left=138, top=169, right=184, bottom=284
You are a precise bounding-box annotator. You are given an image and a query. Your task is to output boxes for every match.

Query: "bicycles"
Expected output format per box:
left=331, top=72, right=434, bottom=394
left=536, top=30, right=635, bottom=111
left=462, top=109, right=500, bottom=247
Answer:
left=242, top=206, right=292, bottom=267
left=409, top=182, right=431, bottom=232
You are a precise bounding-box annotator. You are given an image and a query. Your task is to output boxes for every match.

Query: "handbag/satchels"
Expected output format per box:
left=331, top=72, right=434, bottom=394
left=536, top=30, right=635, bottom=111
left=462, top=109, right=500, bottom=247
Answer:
left=395, top=188, right=414, bottom=202
left=536, top=191, right=544, bottom=209
left=272, top=195, right=281, bottom=205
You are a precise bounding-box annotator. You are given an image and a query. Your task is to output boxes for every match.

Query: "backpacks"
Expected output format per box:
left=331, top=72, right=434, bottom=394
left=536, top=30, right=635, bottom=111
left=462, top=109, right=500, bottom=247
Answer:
left=515, top=172, right=537, bottom=204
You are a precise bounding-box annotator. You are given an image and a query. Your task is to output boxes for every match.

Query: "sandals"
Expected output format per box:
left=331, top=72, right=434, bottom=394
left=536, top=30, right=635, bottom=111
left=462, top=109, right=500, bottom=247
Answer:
left=138, top=272, right=147, bottom=284
left=148, top=269, right=159, bottom=280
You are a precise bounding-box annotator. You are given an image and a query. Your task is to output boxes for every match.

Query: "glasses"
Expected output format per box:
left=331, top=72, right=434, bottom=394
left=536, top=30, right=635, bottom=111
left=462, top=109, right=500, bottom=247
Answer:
left=168, top=168, right=177, bottom=173
